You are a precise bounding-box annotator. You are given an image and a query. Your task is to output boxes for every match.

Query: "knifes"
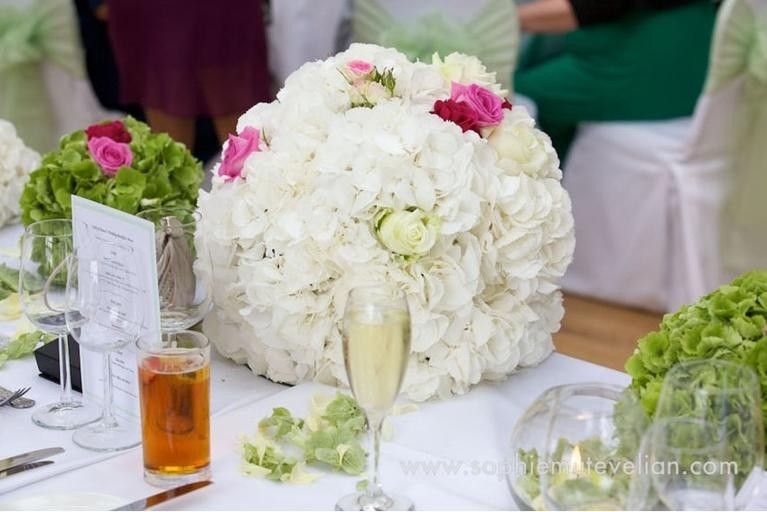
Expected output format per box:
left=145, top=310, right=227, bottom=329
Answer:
left=0, top=446, right=67, bottom=477
left=0, top=458, right=55, bottom=479
left=107, top=478, right=213, bottom=512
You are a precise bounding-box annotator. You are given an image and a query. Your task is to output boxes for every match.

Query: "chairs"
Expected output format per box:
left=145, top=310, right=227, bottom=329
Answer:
left=353, top=0, right=539, bottom=122
left=558, top=0, right=767, bottom=305
left=0, top=0, right=130, bottom=156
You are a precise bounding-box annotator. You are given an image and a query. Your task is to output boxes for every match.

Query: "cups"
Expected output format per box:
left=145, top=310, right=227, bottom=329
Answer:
left=502, top=378, right=657, bottom=512
left=134, top=326, right=215, bottom=490
left=623, top=409, right=739, bottom=512
left=649, top=359, right=766, bottom=512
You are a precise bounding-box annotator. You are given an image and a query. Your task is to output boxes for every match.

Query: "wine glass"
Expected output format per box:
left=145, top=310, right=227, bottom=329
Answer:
left=133, top=203, right=217, bottom=353
left=63, top=233, right=145, bottom=452
left=333, top=283, right=419, bottom=512
left=14, top=218, right=105, bottom=432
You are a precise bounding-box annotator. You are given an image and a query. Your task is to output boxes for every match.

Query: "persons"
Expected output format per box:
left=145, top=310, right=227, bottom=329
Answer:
left=73, top=0, right=277, bottom=156
left=511, top=0, right=722, bottom=166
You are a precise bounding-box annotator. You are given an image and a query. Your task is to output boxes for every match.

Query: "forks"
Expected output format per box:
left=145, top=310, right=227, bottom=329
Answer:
left=0, top=384, right=36, bottom=410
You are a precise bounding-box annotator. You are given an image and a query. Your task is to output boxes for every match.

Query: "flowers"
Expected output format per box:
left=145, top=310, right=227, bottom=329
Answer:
left=0, top=47, right=766, bottom=492
